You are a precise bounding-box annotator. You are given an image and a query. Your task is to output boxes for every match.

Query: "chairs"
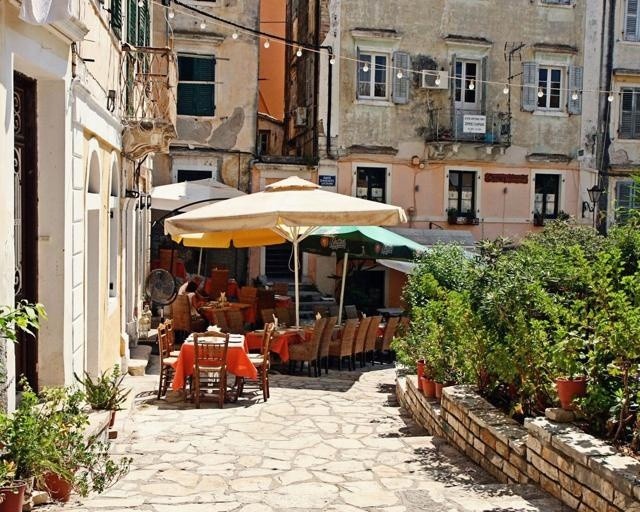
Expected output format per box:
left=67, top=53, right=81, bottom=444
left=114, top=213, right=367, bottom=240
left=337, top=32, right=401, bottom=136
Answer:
left=246, top=304, right=411, bottom=378
left=152, top=318, right=275, bottom=410
left=167, top=266, right=293, bottom=345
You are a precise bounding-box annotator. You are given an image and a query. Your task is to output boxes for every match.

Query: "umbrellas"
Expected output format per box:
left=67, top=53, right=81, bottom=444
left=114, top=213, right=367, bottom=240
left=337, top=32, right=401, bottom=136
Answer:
left=149, top=176, right=247, bottom=277
left=162, top=174, right=409, bottom=332
left=286, top=225, right=428, bottom=326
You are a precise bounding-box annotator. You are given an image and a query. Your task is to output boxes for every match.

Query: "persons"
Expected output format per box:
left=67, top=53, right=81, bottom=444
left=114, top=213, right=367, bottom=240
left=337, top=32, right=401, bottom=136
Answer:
left=224, top=277, right=240, bottom=301
left=184, top=281, right=205, bottom=321
left=176, top=273, right=205, bottom=296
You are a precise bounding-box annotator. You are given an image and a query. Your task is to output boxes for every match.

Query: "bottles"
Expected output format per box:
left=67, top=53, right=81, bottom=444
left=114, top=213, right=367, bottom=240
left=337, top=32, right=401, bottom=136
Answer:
left=139, top=305, right=152, bottom=339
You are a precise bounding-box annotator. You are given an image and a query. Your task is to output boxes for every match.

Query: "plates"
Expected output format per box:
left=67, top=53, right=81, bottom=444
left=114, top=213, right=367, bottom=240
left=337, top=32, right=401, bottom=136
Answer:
left=229, top=334, right=241, bottom=343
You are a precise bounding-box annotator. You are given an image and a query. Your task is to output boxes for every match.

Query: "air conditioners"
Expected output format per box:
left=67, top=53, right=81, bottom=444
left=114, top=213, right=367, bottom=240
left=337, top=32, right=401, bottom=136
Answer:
left=420, top=70, right=448, bottom=90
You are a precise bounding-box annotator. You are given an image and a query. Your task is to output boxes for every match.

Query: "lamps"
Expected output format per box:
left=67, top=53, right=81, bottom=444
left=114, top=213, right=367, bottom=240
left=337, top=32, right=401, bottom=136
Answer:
left=585, top=185, right=603, bottom=218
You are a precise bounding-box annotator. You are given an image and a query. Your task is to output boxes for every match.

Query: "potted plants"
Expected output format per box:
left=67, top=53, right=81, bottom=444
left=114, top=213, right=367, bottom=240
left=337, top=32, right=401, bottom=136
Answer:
left=395, top=225, right=587, bottom=411
left=0, top=298, right=133, bottom=512
